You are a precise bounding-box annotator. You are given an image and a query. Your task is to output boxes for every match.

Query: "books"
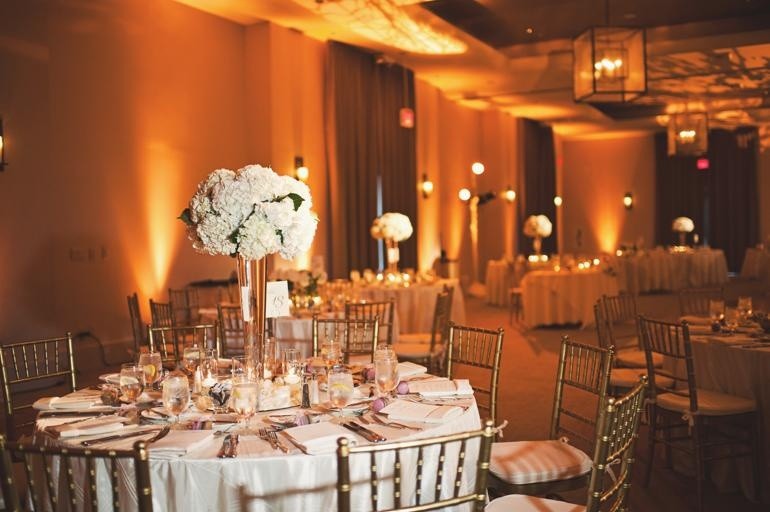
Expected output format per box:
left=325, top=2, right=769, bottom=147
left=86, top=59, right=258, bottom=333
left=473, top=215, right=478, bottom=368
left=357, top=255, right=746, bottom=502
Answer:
left=398, top=360, right=428, bottom=378
left=421, top=379, right=474, bottom=397
left=53, top=398, right=93, bottom=409
left=46, top=422, right=123, bottom=438
left=388, top=408, right=463, bottom=423
left=284, top=422, right=346, bottom=445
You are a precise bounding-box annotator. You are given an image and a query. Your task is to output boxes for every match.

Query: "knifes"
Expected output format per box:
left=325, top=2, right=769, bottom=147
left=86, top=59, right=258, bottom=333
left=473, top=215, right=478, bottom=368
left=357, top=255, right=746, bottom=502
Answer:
left=272, top=425, right=312, bottom=455
left=81, top=429, right=150, bottom=448
left=216, top=432, right=239, bottom=460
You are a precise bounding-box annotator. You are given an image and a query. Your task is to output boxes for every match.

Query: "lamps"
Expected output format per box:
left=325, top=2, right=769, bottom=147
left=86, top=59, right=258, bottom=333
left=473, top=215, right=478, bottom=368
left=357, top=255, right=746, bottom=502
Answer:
left=665, top=94, right=711, bottom=159
left=571, top=0, right=653, bottom=107
left=399, top=71, right=415, bottom=127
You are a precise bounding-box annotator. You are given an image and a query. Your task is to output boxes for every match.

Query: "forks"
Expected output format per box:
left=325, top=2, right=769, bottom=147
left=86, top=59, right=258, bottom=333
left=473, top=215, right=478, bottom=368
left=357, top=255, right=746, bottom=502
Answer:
left=258, top=426, right=289, bottom=454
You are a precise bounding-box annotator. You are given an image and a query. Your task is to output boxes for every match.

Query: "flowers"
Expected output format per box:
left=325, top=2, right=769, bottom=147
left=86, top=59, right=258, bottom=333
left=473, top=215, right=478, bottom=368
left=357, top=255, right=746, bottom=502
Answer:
left=671, top=217, right=695, bottom=233
left=369, top=212, right=413, bottom=242
left=522, top=215, right=553, bottom=238
left=182, top=164, right=320, bottom=263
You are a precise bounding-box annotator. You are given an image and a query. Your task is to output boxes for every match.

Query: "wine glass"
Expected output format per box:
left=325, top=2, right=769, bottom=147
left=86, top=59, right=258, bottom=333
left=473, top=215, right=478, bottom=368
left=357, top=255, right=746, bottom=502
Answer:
left=736, top=295, right=753, bottom=322
left=120, top=341, right=400, bottom=436
left=710, top=298, right=725, bottom=324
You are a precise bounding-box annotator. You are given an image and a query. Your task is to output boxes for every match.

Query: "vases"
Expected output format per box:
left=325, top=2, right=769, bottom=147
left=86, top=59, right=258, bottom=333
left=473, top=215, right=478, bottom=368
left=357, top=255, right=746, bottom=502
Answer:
left=531, top=238, right=542, bottom=254
left=679, top=233, right=686, bottom=245
